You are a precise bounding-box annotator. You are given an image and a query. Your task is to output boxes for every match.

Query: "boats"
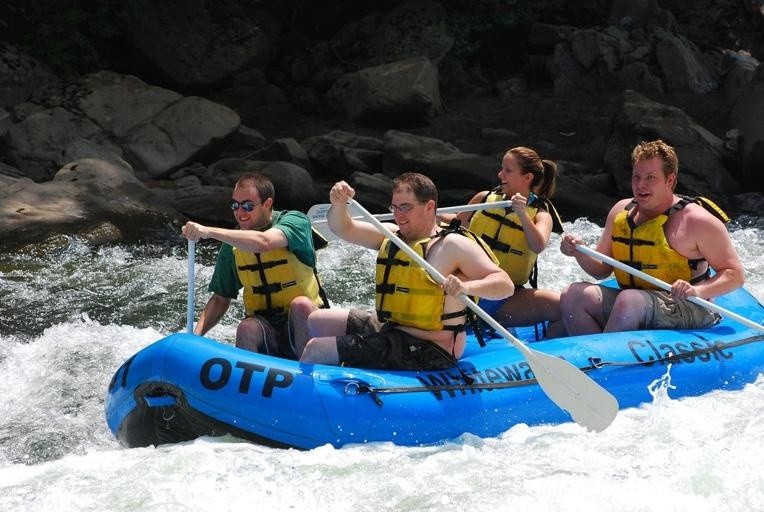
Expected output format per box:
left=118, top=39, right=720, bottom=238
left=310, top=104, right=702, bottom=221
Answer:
left=104, top=267, right=764, bottom=452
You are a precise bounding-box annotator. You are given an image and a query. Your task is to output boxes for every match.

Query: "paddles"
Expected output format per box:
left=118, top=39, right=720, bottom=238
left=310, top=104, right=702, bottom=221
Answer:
left=306, top=200, right=516, bottom=243
left=346, top=193, right=620, bottom=435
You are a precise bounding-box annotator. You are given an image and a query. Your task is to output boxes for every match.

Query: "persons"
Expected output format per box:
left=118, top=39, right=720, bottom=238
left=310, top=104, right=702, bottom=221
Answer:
left=295, top=167, right=514, bottom=374
left=556, top=134, right=745, bottom=333
left=176, top=166, right=333, bottom=361
left=451, top=146, right=577, bottom=339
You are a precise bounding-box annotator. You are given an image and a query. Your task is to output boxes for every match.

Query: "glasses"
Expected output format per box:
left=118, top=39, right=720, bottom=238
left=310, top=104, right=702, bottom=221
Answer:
left=229, top=198, right=263, bottom=213
left=388, top=203, right=415, bottom=214
